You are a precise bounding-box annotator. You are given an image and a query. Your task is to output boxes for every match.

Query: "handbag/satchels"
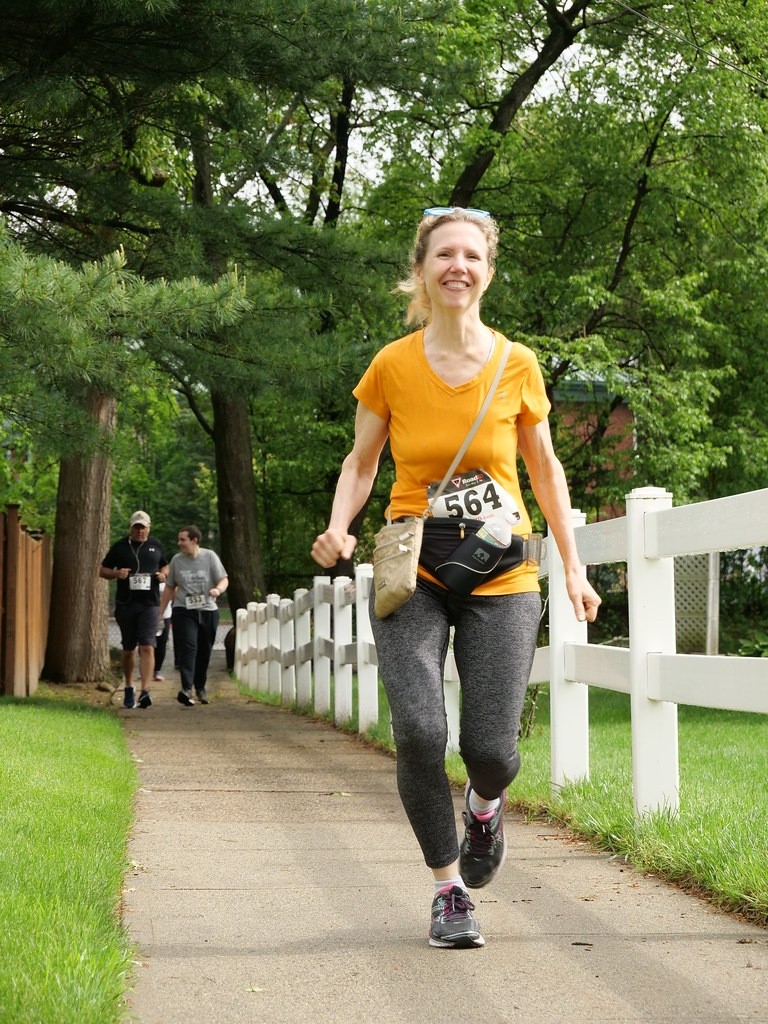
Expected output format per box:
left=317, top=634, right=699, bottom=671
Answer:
left=371, top=516, right=424, bottom=619
left=392, top=516, right=547, bottom=597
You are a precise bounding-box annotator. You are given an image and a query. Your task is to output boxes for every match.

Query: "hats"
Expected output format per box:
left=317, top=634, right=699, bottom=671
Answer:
left=130, top=510, right=151, bottom=528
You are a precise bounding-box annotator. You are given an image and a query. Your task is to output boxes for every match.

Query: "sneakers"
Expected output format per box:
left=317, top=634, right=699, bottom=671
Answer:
left=458, top=777, right=507, bottom=889
left=195, top=687, right=209, bottom=705
left=177, top=689, right=196, bottom=707
left=137, top=690, right=152, bottom=709
left=123, top=685, right=135, bottom=709
left=428, top=884, right=486, bottom=950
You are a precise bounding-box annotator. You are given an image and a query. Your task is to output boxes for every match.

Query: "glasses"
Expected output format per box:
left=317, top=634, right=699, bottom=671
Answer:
left=424, top=206, right=490, bottom=219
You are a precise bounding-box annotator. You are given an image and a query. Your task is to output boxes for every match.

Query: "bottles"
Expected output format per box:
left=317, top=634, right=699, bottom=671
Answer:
left=476, top=512, right=519, bottom=549
left=156, top=620, right=164, bottom=636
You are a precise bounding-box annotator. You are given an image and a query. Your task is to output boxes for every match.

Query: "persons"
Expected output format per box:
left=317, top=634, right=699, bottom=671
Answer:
left=310, top=203, right=602, bottom=950
left=159, top=524, right=230, bottom=706
left=100, top=510, right=170, bottom=709
left=137, top=555, right=173, bottom=682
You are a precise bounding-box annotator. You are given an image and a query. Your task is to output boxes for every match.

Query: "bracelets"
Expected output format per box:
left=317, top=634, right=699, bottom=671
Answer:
left=162, top=572, right=168, bottom=580
left=215, top=587, right=222, bottom=594
